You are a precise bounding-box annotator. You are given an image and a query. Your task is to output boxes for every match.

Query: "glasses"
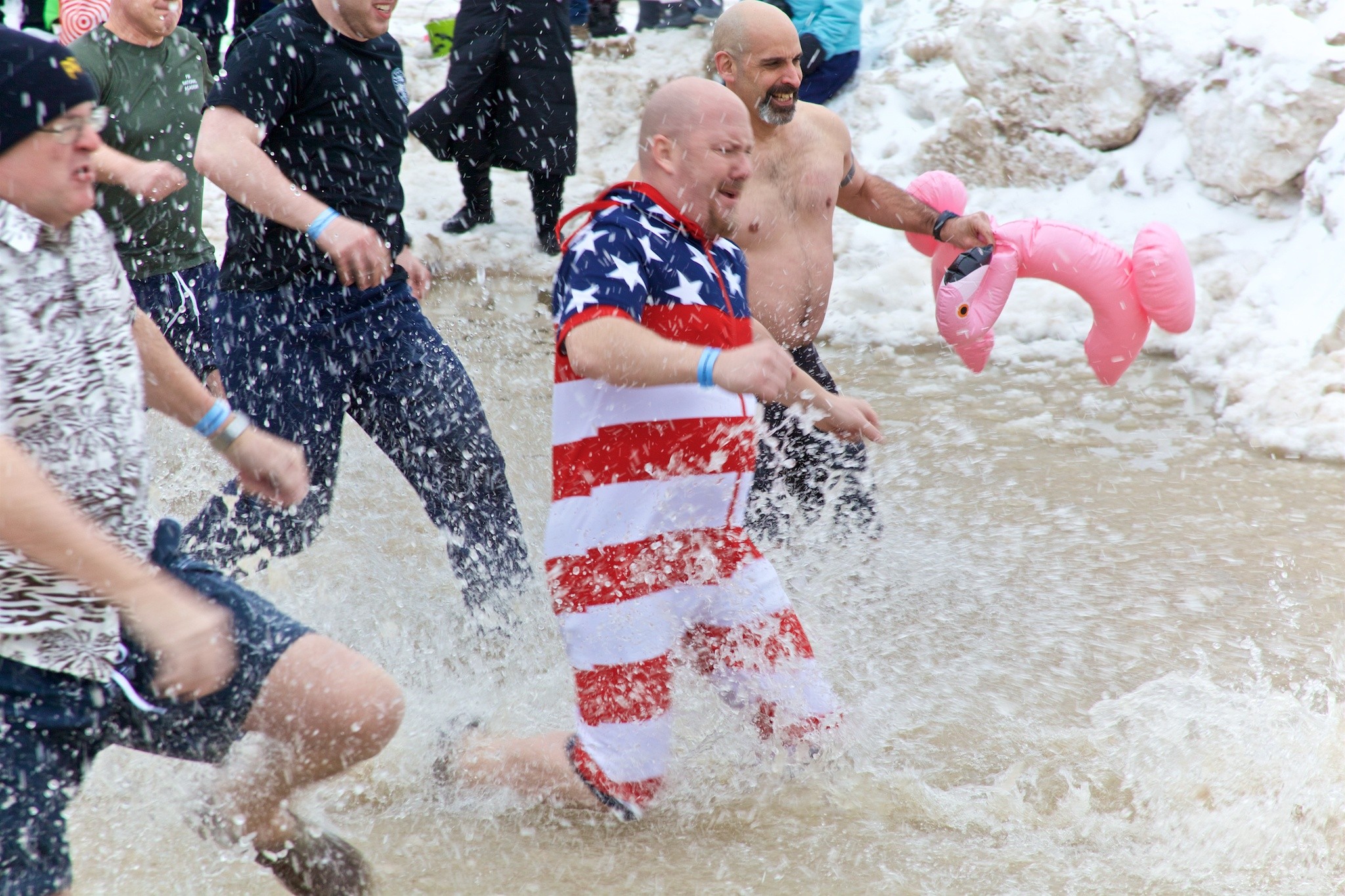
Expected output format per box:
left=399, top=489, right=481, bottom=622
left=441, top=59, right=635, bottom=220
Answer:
left=39, top=104, right=109, bottom=146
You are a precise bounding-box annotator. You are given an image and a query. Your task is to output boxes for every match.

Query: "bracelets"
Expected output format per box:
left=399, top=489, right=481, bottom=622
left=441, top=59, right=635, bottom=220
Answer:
left=193, top=398, right=230, bottom=436
left=933, top=210, right=959, bottom=241
left=211, top=414, right=250, bottom=451
left=697, top=346, right=721, bottom=386
left=306, top=206, right=341, bottom=242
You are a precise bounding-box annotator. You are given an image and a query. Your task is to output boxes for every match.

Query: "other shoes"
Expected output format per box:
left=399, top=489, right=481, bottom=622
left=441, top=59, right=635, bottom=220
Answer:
left=692, top=0, right=723, bottom=24
left=591, top=25, right=626, bottom=37
left=441, top=206, right=493, bottom=233
left=571, top=33, right=585, bottom=51
left=538, top=222, right=567, bottom=255
left=195, top=790, right=368, bottom=896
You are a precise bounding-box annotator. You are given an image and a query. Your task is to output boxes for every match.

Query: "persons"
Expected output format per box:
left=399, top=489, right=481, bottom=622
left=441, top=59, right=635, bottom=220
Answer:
left=0, top=23, right=408, bottom=896
left=433, top=77, right=885, bottom=823
left=407, top=0, right=578, bottom=255
left=785, top=0, right=863, bottom=103
left=626, top=0, right=994, bottom=534
left=178, top=0, right=275, bottom=77
left=183, top=0, right=533, bottom=648
left=568, top=0, right=626, bottom=38
left=67, top=0, right=220, bottom=386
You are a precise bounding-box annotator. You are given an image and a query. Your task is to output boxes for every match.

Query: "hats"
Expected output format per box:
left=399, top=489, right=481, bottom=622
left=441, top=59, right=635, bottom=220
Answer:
left=0, top=24, right=100, bottom=154
